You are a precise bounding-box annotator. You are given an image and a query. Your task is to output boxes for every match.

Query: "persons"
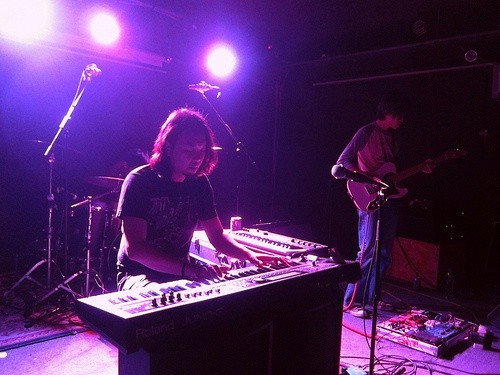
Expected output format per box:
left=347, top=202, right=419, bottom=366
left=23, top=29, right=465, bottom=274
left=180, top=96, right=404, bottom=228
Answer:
left=114, top=108, right=264, bottom=291
left=336, top=95, right=439, bottom=318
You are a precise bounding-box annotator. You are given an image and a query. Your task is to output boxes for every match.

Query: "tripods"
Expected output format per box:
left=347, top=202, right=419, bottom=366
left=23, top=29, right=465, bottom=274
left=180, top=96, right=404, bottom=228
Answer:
left=2, top=77, right=121, bottom=310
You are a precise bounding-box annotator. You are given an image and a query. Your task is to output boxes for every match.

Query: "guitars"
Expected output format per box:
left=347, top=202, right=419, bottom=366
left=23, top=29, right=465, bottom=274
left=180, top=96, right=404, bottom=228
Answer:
left=346, top=146, right=469, bottom=214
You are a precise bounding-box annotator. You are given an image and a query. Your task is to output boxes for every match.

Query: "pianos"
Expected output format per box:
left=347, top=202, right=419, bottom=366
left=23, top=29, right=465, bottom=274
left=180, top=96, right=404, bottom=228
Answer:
left=191, top=228, right=329, bottom=264
left=75, top=253, right=364, bottom=356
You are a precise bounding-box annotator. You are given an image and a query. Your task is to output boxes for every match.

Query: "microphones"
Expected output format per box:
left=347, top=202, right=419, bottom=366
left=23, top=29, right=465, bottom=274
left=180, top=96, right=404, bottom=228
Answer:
left=85, top=63, right=101, bottom=77
left=188, top=85, right=220, bottom=89
left=331, top=164, right=390, bottom=188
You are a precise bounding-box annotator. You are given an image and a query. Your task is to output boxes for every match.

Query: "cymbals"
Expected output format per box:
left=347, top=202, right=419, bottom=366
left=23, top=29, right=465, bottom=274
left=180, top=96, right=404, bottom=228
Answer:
left=84, top=173, right=125, bottom=193
left=26, top=137, right=89, bottom=161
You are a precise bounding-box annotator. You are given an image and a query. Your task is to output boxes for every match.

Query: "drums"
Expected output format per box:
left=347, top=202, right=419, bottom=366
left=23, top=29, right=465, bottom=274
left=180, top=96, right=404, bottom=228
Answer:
left=105, top=201, right=122, bottom=237
left=64, top=197, right=107, bottom=256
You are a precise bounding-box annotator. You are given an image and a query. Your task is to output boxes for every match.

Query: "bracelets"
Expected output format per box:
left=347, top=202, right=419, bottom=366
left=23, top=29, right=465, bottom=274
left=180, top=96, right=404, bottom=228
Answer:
left=182, top=261, right=188, bottom=277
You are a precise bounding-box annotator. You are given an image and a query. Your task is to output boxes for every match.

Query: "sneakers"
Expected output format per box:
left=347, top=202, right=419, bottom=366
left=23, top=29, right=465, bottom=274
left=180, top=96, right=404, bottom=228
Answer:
left=343, top=304, right=368, bottom=317
left=365, top=300, right=394, bottom=311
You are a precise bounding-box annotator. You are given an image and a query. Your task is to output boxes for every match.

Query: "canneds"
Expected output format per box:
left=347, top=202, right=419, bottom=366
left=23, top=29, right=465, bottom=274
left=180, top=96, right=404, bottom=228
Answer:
left=231, top=217, right=242, bottom=231
left=413, top=277, right=420, bottom=289
